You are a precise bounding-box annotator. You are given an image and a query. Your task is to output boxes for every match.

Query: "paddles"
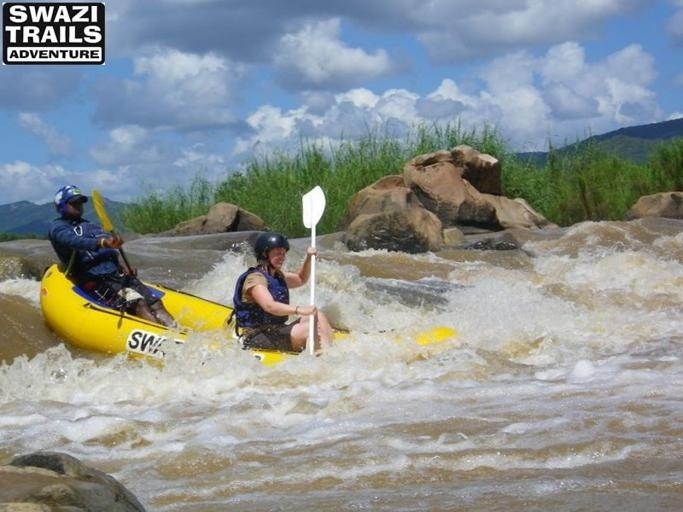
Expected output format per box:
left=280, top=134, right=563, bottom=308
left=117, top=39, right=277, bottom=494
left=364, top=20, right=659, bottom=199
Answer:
left=92, top=190, right=134, bottom=276
left=301, top=185, right=326, bottom=356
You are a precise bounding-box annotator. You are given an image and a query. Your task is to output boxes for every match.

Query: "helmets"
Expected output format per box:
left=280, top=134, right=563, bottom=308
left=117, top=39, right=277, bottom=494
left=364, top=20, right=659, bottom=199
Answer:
left=255, top=232, right=290, bottom=261
left=53, top=184, right=88, bottom=214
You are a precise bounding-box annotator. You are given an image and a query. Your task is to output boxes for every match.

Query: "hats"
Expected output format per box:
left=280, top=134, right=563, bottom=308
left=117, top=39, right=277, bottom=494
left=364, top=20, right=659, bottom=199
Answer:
left=68, top=195, right=87, bottom=204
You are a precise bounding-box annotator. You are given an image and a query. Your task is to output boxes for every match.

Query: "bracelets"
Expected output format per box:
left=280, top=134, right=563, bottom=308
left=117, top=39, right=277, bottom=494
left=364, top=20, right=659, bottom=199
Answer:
left=100, top=237, right=105, bottom=248
left=295, top=306, right=299, bottom=314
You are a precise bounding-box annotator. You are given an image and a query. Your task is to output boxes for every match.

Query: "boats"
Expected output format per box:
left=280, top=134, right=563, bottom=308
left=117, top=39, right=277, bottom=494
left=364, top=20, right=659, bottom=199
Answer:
left=39, top=263, right=459, bottom=383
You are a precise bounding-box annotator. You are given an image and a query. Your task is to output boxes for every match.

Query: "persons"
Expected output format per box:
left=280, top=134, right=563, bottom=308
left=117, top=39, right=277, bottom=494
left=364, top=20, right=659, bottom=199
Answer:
left=46, top=183, right=178, bottom=329
left=232, top=231, right=337, bottom=354
